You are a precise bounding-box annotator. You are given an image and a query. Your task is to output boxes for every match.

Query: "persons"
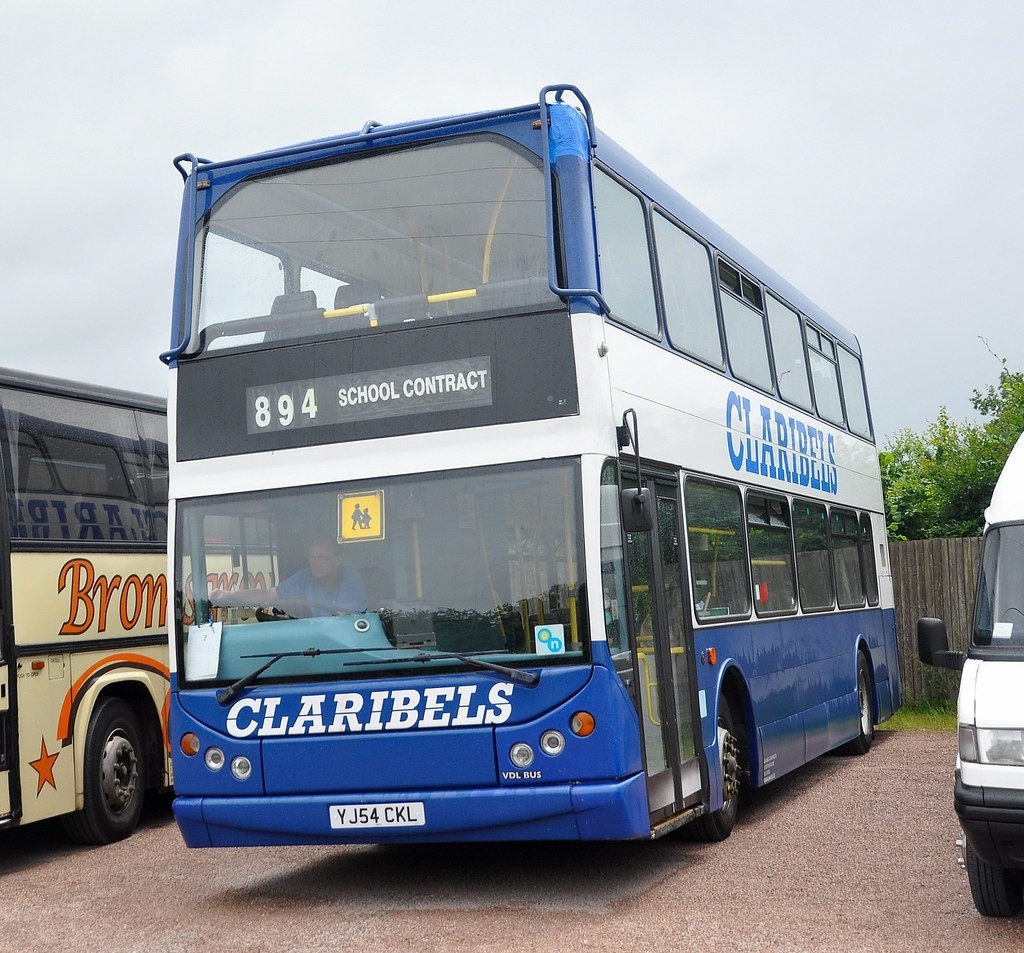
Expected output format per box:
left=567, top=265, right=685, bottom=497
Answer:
left=210, top=536, right=367, bottom=618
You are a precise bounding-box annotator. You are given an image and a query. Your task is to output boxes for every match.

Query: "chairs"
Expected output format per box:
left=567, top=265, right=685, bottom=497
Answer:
left=258, top=284, right=402, bottom=343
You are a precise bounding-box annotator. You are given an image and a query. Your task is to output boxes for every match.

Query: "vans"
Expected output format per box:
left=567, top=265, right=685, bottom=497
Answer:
left=918, top=428, right=1022, bottom=913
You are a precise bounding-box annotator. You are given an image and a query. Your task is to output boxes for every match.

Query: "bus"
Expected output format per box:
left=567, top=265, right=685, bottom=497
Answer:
left=161, top=77, right=905, bottom=871
left=0, top=366, right=177, bottom=847
